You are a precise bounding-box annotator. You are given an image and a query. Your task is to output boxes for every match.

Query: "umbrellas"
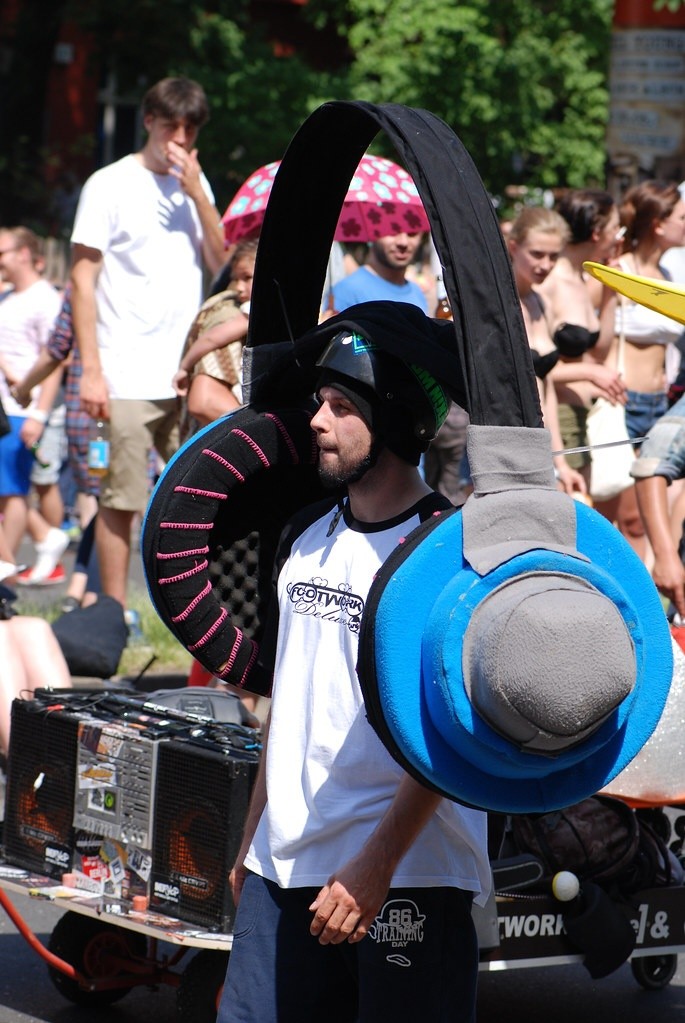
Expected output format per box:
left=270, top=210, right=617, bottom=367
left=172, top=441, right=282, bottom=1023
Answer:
left=219, top=154, right=430, bottom=309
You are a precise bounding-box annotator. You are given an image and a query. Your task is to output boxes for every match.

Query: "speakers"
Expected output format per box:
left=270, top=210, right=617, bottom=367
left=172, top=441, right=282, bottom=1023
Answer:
left=0, top=694, right=265, bottom=934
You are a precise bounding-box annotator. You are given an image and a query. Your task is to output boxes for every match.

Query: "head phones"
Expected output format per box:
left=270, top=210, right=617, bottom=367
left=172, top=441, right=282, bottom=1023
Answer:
left=138, top=100, right=675, bottom=814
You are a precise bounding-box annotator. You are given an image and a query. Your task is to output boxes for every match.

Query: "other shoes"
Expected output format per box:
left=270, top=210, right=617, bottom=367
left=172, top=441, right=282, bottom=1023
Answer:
left=27, top=527, right=70, bottom=585
left=124, top=609, right=143, bottom=646
left=62, top=597, right=80, bottom=615
left=16, top=563, right=65, bottom=586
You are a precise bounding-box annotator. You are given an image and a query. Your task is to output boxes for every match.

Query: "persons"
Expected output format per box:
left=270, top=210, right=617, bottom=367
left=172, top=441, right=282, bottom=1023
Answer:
left=502, top=177, right=685, bottom=620
left=216, top=300, right=494, bottom=1023
left=0, top=79, right=431, bottom=646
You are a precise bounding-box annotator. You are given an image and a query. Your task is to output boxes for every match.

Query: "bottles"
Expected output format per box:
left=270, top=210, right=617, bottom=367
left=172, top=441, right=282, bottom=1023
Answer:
left=434, top=272, right=453, bottom=320
left=87, top=419, right=111, bottom=478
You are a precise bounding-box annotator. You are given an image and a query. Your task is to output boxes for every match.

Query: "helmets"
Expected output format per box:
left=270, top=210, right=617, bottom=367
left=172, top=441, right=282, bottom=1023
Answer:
left=314, top=329, right=453, bottom=453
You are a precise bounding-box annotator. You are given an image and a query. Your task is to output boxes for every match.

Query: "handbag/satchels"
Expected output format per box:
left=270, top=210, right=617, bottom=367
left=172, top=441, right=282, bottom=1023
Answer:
left=585, top=395, right=636, bottom=502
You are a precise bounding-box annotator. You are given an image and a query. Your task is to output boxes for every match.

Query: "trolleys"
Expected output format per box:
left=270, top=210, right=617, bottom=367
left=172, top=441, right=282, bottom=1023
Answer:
left=0, top=855, right=685, bottom=1023
